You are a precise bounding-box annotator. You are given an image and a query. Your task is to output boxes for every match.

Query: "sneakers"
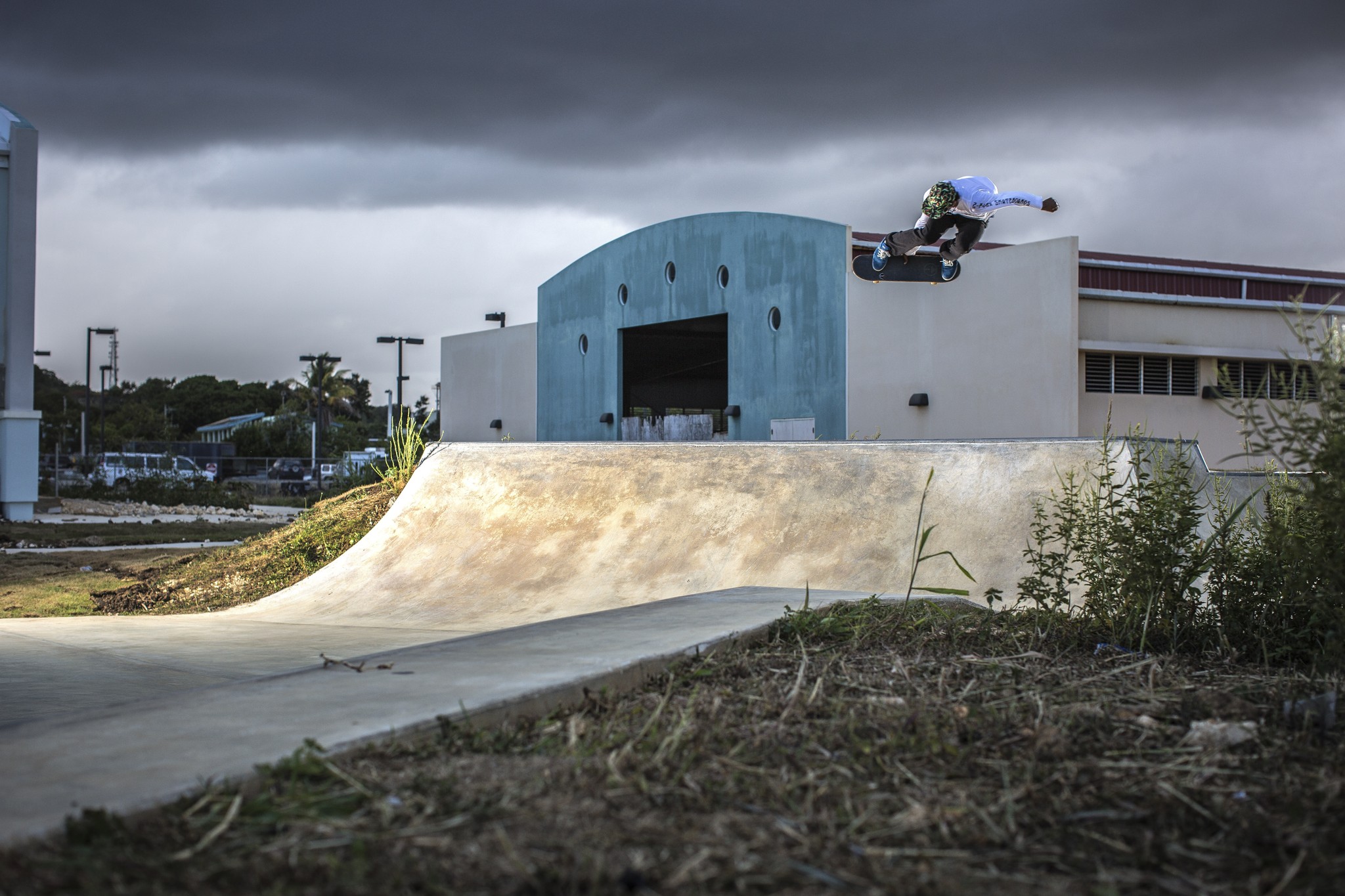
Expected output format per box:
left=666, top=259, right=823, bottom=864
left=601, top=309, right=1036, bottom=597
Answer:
left=872, top=234, right=891, bottom=271
left=940, top=257, right=958, bottom=280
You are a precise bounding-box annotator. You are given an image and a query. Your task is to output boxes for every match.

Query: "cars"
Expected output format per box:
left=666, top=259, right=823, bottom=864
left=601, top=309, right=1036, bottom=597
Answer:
left=267, top=458, right=305, bottom=481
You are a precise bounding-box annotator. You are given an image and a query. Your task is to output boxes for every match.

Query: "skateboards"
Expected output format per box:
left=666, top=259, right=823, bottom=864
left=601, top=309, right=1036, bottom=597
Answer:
left=852, top=254, right=961, bottom=284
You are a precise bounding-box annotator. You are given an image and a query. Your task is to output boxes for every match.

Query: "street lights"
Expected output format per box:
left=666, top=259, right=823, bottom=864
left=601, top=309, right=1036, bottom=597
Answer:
left=485, top=311, right=506, bottom=328
left=376, top=336, right=424, bottom=435
left=99, top=364, right=113, bottom=454
left=299, top=355, right=342, bottom=490
left=84, top=327, right=114, bottom=475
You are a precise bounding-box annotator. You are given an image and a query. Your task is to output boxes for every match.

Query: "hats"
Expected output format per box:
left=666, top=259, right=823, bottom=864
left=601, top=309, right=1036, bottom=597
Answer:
left=921, top=182, right=956, bottom=220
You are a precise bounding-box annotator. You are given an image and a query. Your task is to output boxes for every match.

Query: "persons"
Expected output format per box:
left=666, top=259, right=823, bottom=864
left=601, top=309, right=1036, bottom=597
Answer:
left=872, top=175, right=1060, bottom=282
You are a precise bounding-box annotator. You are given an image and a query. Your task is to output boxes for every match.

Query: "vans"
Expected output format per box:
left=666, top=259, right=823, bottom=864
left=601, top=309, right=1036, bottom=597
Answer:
left=320, top=464, right=337, bottom=481
left=93, top=451, right=214, bottom=493
